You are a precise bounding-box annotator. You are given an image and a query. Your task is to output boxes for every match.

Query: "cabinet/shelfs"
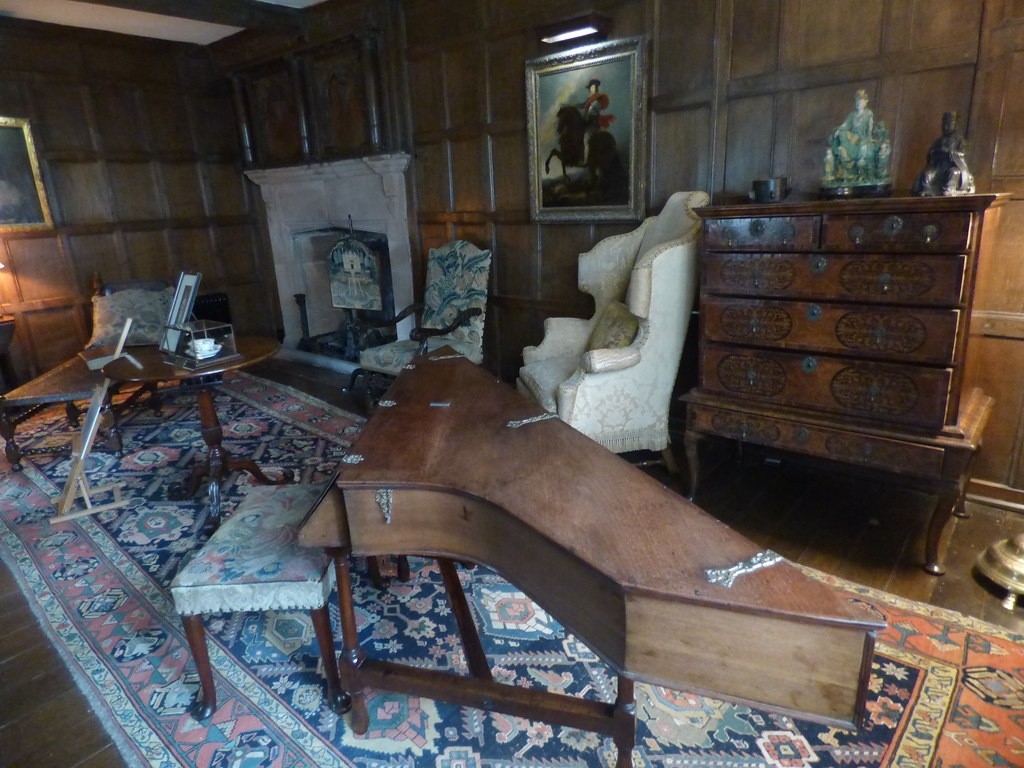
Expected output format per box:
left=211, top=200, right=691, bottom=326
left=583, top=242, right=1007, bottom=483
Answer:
left=677, top=191, right=1015, bottom=577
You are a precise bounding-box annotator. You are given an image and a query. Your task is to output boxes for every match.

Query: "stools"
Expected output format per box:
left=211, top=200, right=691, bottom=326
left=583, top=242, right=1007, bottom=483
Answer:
left=170, top=484, right=388, bottom=719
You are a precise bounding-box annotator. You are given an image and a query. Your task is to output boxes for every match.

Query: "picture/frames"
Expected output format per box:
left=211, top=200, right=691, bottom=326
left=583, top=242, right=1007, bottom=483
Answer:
left=524, top=34, right=648, bottom=224
left=0, top=114, right=54, bottom=233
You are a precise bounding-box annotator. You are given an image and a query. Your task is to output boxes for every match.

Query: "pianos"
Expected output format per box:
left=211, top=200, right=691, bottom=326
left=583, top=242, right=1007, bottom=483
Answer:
left=290, top=346, right=891, bottom=767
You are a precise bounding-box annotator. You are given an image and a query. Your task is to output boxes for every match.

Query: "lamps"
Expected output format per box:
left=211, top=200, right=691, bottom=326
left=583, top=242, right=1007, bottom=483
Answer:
left=537, top=16, right=607, bottom=46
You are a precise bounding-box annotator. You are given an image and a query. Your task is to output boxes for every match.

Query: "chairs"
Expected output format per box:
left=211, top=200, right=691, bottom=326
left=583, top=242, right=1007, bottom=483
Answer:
left=516, top=190, right=710, bottom=496
left=358, top=240, right=493, bottom=417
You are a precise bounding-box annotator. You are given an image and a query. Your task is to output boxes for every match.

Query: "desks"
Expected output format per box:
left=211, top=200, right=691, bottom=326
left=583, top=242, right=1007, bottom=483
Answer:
left=0, top=356, right=163, bottom=472
left=102, top=334, right=280, bottom=530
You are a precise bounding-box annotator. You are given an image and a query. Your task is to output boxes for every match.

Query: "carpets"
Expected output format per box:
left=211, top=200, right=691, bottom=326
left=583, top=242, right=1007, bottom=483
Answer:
left=0, top=369, right=1024, bottom=768
